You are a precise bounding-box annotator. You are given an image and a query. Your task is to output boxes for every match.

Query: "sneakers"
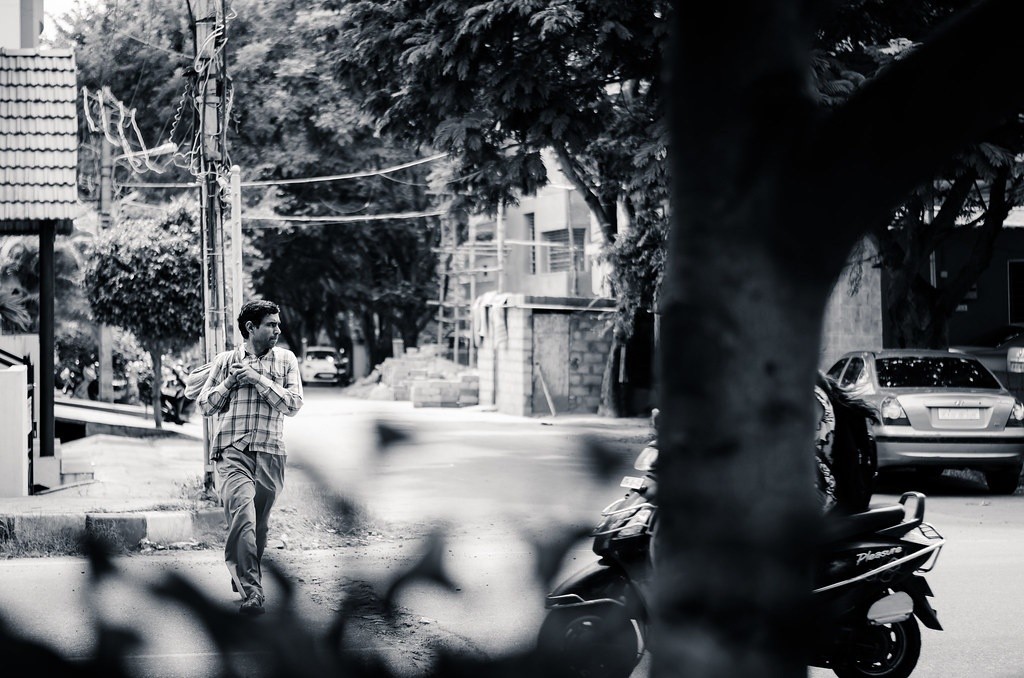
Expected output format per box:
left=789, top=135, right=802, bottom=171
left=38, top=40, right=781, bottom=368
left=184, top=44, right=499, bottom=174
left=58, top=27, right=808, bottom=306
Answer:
left=240, top=592, right=264, bottom=614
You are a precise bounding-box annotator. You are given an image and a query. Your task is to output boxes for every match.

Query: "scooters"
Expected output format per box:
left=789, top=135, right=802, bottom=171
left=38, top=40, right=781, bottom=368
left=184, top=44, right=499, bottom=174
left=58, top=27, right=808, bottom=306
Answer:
left=60, top=356, right=199, bottom=426
left=534, top=408, right=945, bottom=678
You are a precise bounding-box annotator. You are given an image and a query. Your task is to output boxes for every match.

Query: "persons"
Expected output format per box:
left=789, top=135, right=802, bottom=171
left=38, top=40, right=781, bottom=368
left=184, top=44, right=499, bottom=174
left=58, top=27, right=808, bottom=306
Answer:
left=814, top=368, right=883, bottom=518
left=196, top=300, right=303, bottom=612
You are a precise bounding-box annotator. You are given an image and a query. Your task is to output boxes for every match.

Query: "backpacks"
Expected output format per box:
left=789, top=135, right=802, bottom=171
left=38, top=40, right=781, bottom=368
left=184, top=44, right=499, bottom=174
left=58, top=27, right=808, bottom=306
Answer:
left=813, top=381, right=878, bottom=510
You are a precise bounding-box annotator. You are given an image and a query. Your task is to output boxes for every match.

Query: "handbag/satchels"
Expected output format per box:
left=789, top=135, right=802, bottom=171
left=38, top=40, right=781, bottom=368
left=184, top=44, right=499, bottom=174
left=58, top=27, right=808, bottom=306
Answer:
left=185, top=350, right=241, bottom=399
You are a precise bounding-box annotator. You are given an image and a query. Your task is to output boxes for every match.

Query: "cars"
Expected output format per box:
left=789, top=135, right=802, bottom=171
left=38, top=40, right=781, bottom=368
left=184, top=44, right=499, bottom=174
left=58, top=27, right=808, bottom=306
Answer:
left=300, top=346, right=341, bottom=385
left=826, top=349, right=1024, bottom=494
left=948, top=323, right=1024, bottom=390
